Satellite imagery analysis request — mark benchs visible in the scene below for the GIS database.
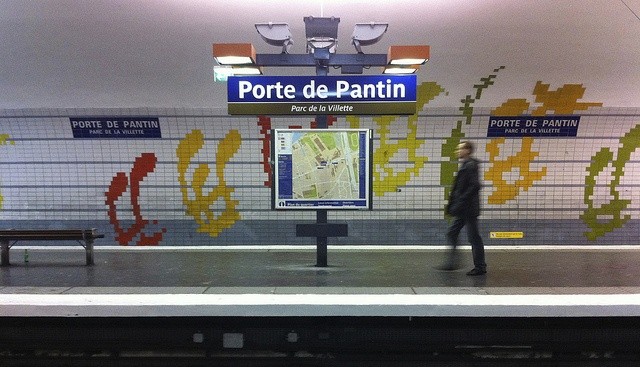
[0,228,105,265]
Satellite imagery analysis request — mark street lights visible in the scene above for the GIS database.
[213,42,428,268]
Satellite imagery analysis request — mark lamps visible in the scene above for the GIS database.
[351,21,389,53]
[387,45,431,65]
[213,64,263,81]
[254,23,293,52]
[213,43,256,65]
[382,64,419,74]
[303,16,341,54]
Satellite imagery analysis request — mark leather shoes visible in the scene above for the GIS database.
[467,269,487,276]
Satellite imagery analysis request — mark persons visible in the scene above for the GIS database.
[434,140,488,276]
